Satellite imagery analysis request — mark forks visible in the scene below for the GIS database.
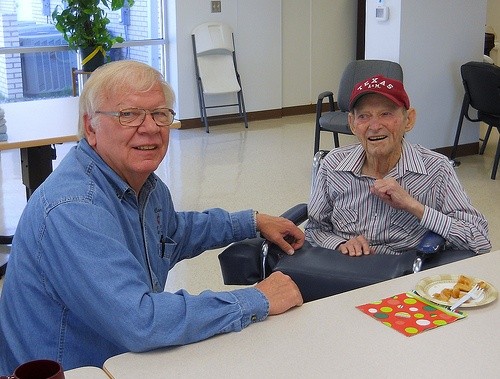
[450,284,484,311]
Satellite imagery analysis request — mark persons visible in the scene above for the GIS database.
[0,59,304,376]
[304,75,492,256]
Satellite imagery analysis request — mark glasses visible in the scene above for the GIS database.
[95,107,177,127]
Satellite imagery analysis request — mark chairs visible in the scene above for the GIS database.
[192,23,249,133]
[314,60,404,155]
[452,60,500,179]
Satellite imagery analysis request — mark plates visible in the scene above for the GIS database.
[415,274,499,308]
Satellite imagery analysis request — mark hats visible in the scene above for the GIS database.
[348,75,410,113]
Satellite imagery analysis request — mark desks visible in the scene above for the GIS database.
[0,96,181,281]
[102,248,500,379]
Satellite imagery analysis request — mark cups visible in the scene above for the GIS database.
[0,359,65,379]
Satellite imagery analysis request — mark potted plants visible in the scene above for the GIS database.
[52,0,136,71]
